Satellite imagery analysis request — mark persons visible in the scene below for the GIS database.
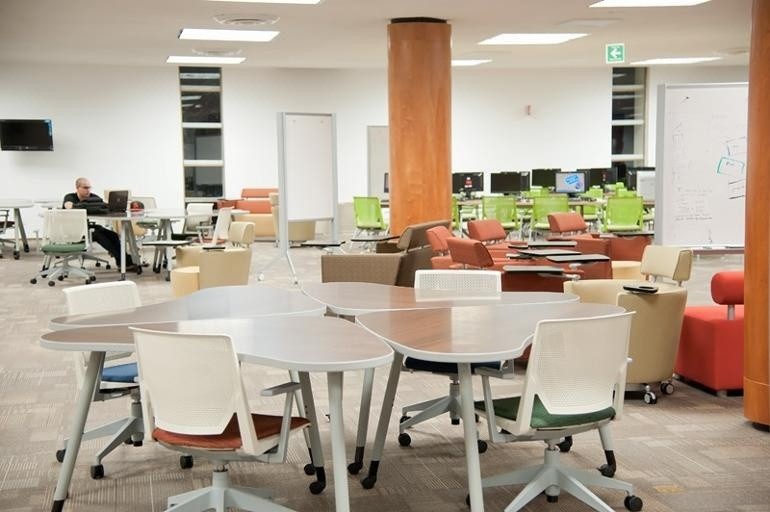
[61,178,132,271]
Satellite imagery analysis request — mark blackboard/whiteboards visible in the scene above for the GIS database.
[654,82,749,253]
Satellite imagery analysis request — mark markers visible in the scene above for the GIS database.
[702,246,713,249]
[725,246,745,248]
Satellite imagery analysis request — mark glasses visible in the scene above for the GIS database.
[77,184,93,190]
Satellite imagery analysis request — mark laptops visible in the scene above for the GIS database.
[98,190,129,214]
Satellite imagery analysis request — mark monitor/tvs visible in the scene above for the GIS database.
[577,169,617,193]
[384,173,389,193]
[501,171,530,191]
[453,172,484,200]
[0,119,55,152]
[555,171,586,194]
[490,172,521,195]
[637,171,656,199]
[626,167,655,189]
[532,168,561,188]
